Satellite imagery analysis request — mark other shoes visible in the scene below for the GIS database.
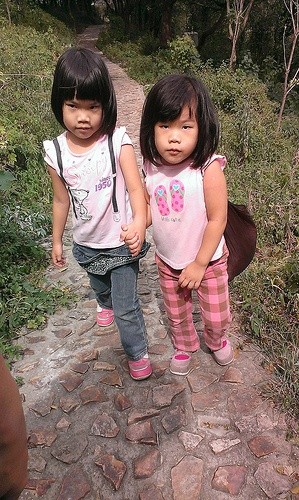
[211,339,234,366]
[170,349,192,375]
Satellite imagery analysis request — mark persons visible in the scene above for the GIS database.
[42,47,152,381]
[118,73,235,377]
[1,351,32,500]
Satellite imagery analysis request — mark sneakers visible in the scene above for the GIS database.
[127,351,152,380]
[96,303,114,326]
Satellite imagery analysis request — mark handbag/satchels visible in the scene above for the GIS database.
[224,200,258,282]
